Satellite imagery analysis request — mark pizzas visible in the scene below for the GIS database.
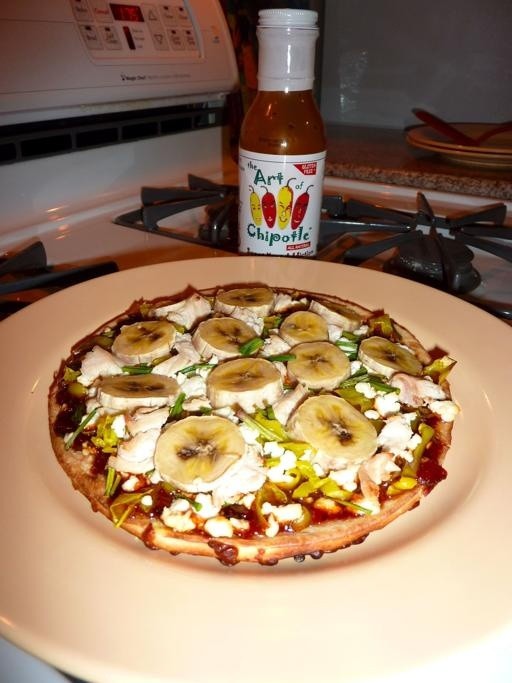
[47,281,461,569]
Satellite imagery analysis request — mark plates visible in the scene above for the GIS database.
[3,254,509,683]
[404,122,511,169]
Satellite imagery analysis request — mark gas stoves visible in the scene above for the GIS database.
[0,164,512,319]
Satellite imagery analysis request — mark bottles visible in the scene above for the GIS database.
[238,7,330,255]
